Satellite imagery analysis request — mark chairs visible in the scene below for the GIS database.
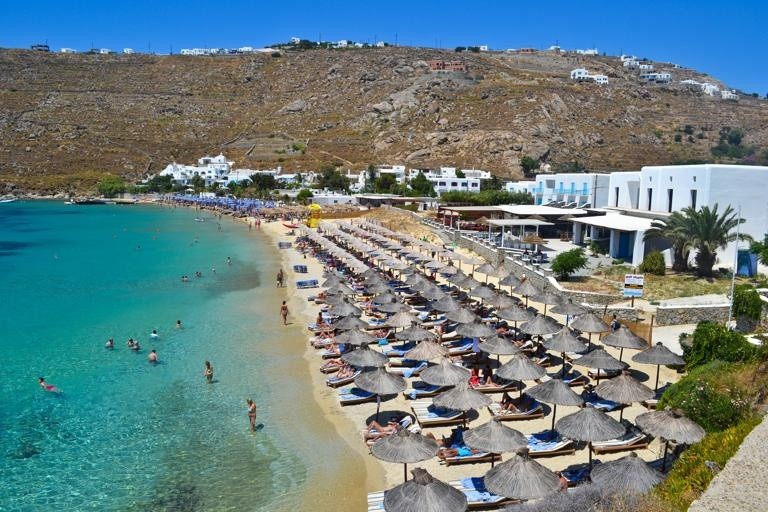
[296,272,680,511]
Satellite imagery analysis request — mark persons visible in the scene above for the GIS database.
[150,330,160,339]
[105,339,113,347]
[126,338,133,346]
[149,350,158,360]
[39,377,63,393]
[132,341,140,350]
[176,195,287,433]
